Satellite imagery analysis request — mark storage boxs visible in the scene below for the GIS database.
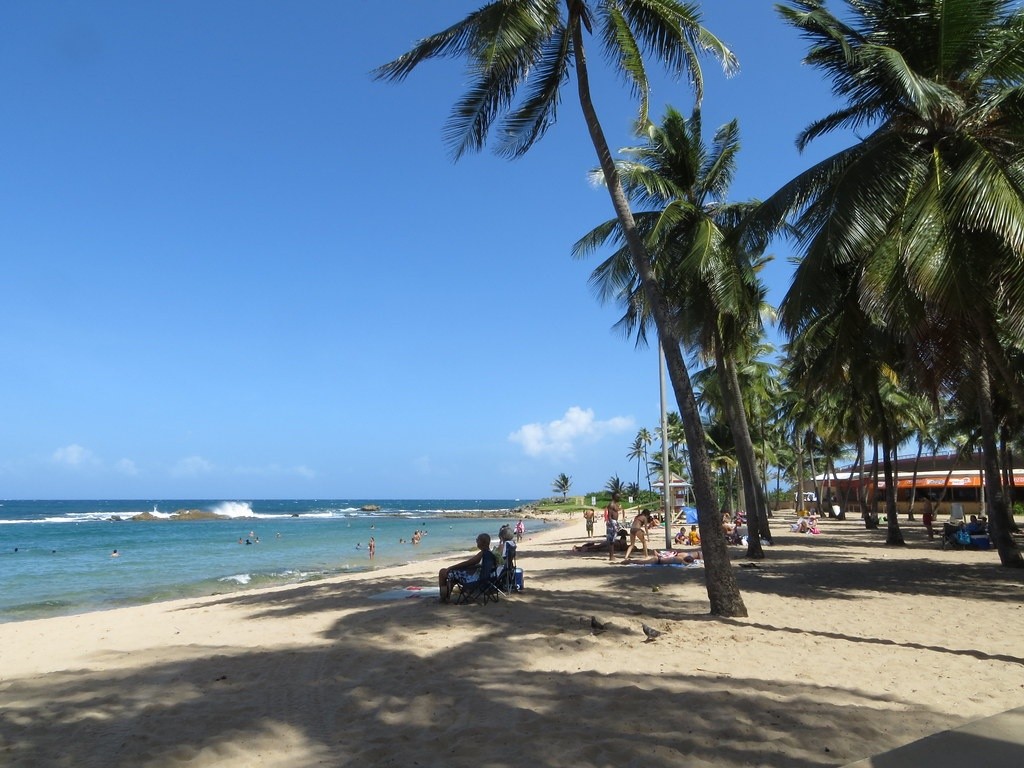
[970,535,989,549]
[513,568,524,590]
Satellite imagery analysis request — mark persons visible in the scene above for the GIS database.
[572,493,821,565]
[922,497,989,548]
[110,519,524,559]
[438,524,517,603]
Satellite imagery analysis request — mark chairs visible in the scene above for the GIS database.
[496,542,517,595]
[455,547,500,607]
[940,522,963,551]
[976,521,991,534]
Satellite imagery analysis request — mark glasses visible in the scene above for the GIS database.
[476,538,479,541]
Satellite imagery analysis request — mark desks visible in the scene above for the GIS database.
[848,504,872,513]
[880,504,900,515]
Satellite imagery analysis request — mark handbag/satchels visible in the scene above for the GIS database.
[514,568,524,590]
[956,525,970,545]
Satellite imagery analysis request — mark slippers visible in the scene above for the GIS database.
[405,594,420,598]
